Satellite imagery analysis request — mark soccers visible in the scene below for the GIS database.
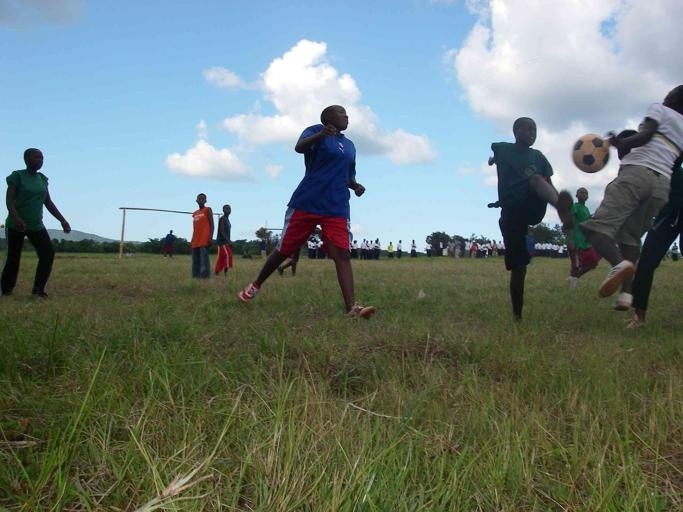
[573,134,609,173]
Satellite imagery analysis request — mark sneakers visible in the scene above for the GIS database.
[625,314,647,329]
[597,259,636,299]
[343,302,376,320]
[557,192,574,229]
[611,292,634,311]
[238,282,261,304]
[577,267,589,278]
[277,266,283,275]
[571,266,579,277]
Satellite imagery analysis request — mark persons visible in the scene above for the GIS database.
[1,148,71,297]
[672,243,679,261]
[487,117,574,323]
[238,105,377,319]
[566,188,602,280]
[387,242,393,258]
[437,238,505,258]
[410,240,417,257]
[308,232,381,261]
[214,205,233,276]
[579,84,683,311]
[191,193,214,278]
[264,232,282,255]
[525,230,535,263]
[279,207,301,275]
[164,230,174,258]
[397,240,402,258]
[618,131,683,329]
[533,241,570,258]
[426,241,433,257]
